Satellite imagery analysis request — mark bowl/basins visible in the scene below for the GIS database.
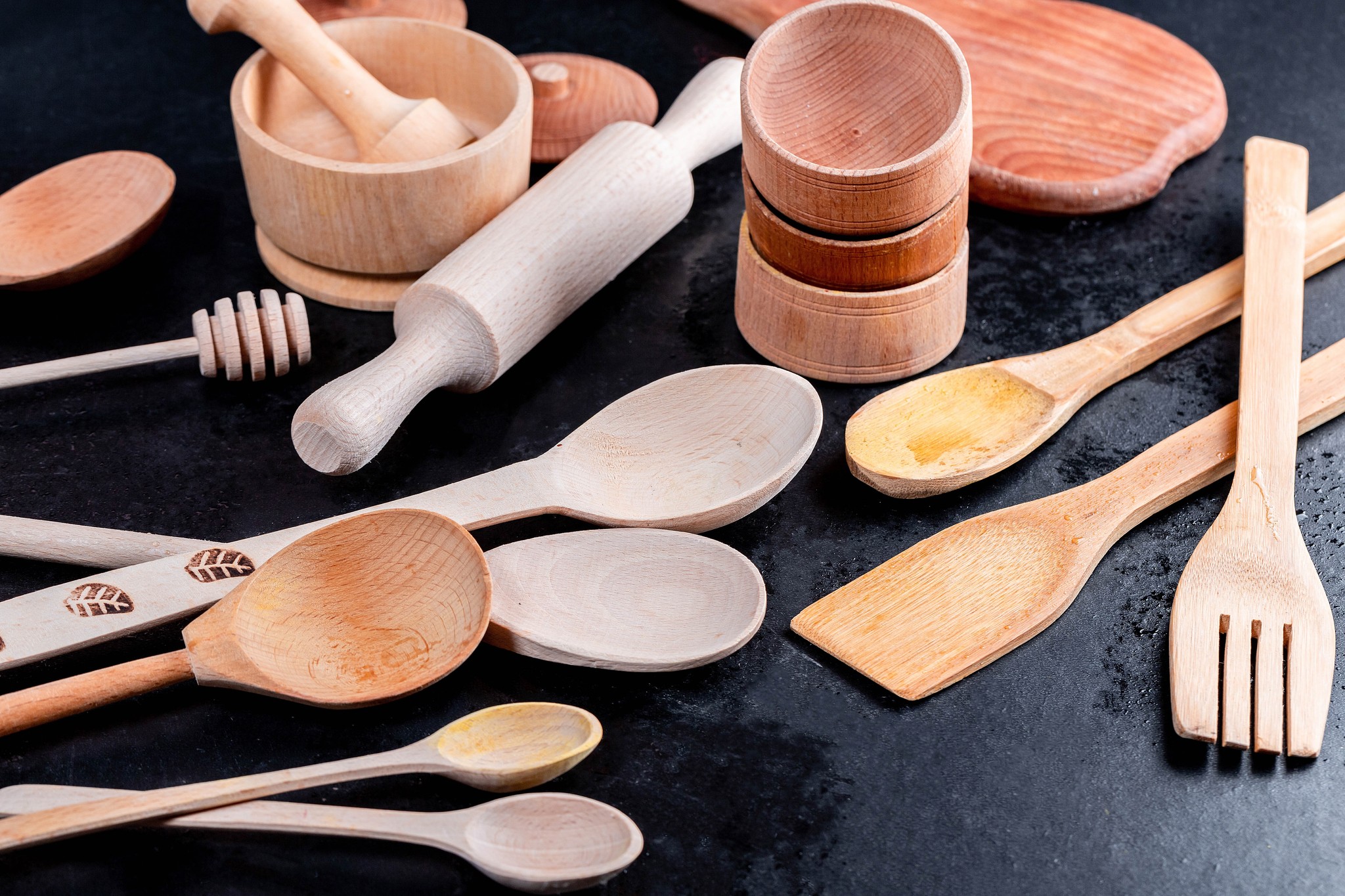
[228,16,534,275]
[735,1,972,384]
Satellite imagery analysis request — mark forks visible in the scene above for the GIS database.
[1170,133,1336,758]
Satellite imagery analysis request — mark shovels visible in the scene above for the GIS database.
[790,339,1345,703]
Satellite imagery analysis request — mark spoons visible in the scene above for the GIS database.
[843,187,1344,504]
[0,361,826,894]
